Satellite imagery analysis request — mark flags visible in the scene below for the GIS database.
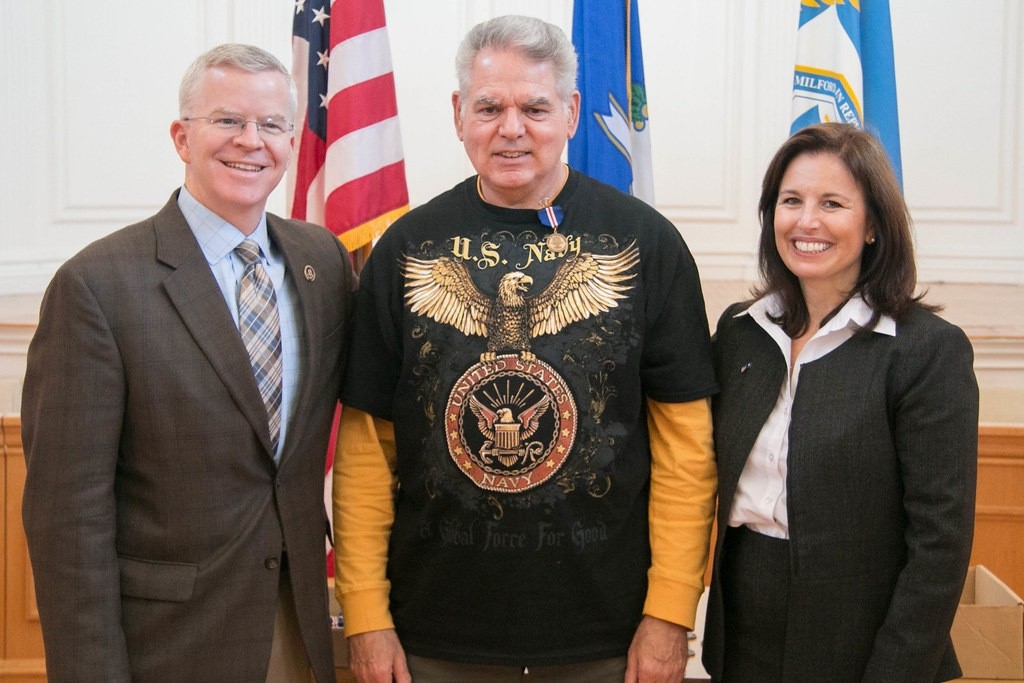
[790,0,905,198]
[568,0,655,208]
[284,0,410,272]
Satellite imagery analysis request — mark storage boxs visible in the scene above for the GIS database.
[949,565,1023,683]
[328,584,349,667]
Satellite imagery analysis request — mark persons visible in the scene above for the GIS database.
[701,123,980,683]
[20,42,355,683]
[331,16,729,683]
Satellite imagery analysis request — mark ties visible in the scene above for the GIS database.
[232,239,283,469]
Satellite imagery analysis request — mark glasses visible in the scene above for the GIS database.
[182,109,294,136]
